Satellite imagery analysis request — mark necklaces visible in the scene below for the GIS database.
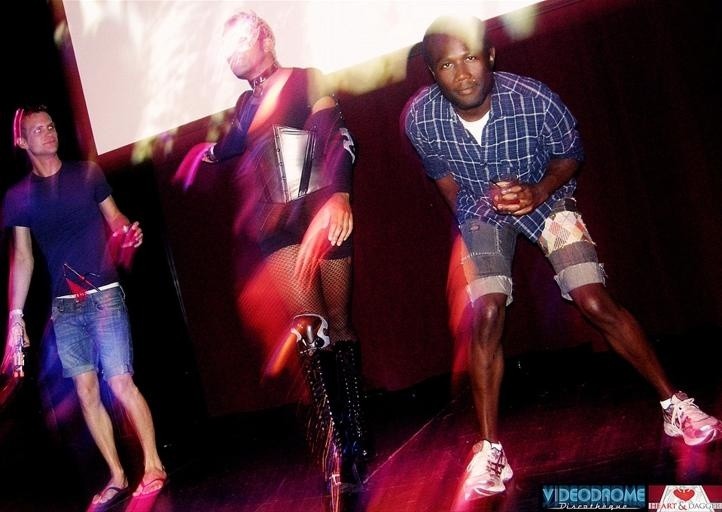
[250,60,281,98]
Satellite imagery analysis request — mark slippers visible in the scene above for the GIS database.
[93,485,129,506]
[135,471,166,499]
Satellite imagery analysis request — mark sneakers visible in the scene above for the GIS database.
[463,440,513,501]
[660,392,722,446]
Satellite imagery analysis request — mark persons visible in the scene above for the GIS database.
[183,8,369,512]
[397,4,721,505]
[2,101,167,509]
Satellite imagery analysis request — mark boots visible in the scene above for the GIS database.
[300,338,376,512]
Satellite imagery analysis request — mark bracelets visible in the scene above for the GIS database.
[203,145,217,162]
[8,308,24,319]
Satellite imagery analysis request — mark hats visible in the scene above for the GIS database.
[13,109,24,146]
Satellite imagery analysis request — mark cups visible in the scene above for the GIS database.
[488,173,519,215]
